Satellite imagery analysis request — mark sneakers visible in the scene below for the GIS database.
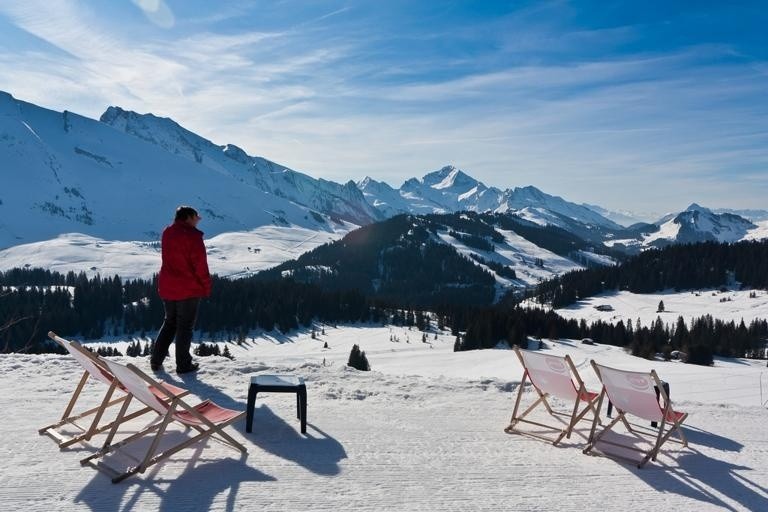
[151,362,162,371]
[175,362,200,375]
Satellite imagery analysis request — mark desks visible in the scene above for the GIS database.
[600,380,672,430]
[242,372,309,435]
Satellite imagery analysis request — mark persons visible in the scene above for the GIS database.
[150,204,214,375]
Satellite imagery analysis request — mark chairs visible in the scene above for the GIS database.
[502,341,602,447]
[80,350,247,482]
[579,356,691,469]
[32,328,207,454]
